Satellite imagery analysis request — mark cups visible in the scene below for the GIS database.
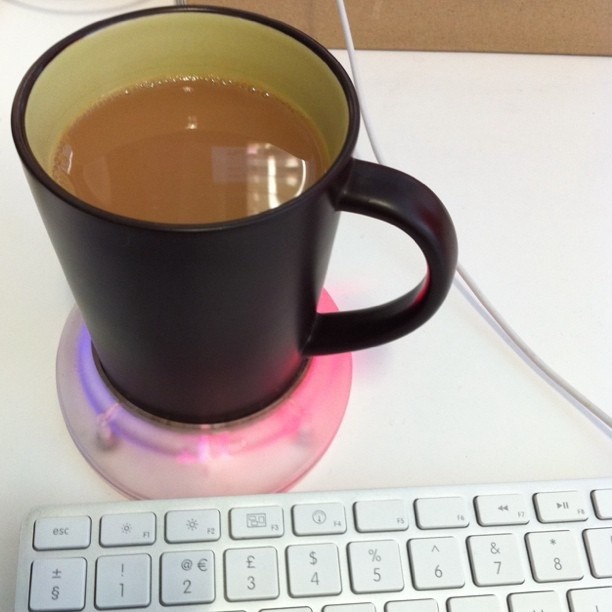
[12,5,456,422]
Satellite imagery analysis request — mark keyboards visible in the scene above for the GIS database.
[12,477,610,612]
